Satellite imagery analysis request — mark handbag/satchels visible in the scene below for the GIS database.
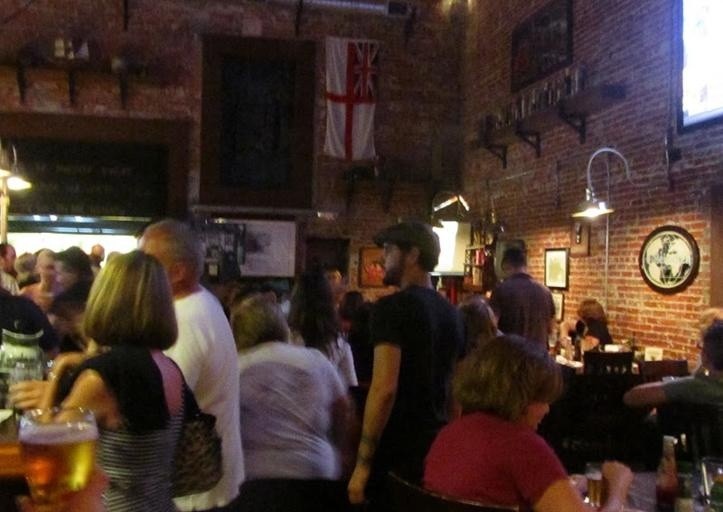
[168,414,222,498]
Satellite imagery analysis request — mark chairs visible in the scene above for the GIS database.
[366,468,525,511]
[541,342,722,475]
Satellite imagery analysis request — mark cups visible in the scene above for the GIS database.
[10,358,43,383]
[586,458,609,508]
[701,456,723,510]
[15,406,102,502]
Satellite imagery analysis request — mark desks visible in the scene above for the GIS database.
[583,467,721,512]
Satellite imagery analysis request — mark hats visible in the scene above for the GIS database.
[373,222,439,259]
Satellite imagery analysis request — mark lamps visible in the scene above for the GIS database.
[426,190,499,249]
[570,146,668,220]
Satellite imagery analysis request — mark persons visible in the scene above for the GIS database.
[621,308,723,473]
[203,220,635,510]
[2,219,247,510]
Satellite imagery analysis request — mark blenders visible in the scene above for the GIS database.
[201,217,247,274]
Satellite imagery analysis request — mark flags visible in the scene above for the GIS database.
[325,35,380,163]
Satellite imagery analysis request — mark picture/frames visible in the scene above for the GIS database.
[637,225,700,295]
[356,244,389,289]
[505,0,571,94]
[196,30,318,213]
[542,246,570,291]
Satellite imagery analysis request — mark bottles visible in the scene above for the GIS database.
[549,329,581,362]
[674,430,697,508]
[655,434,681,510]
[485,63,583,132]
